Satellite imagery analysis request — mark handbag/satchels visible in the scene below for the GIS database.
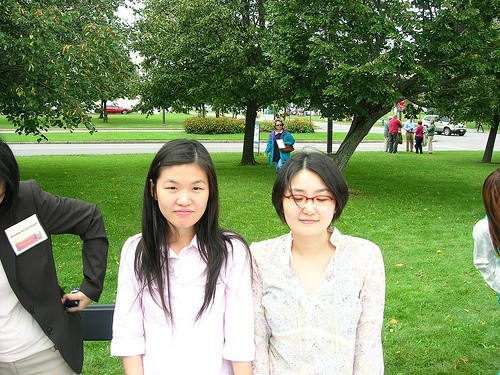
[281,145,294,152]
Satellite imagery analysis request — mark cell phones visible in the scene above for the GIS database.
[64,292,79,307]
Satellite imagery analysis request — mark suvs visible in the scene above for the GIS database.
[422,115,466,136]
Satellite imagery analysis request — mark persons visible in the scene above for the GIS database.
[249,151,386,375]
[0,139,109,375]
[411,120,424,154]
[473,168,500,294]
[388,114,402,153]
[264,120,295,175]
[404,116,414,153]
[110,139,255,375]
[476,122,482,131]
[426,118,436,154]
[384,117,393,152]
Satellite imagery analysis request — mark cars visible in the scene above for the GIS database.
[95,105,132,115]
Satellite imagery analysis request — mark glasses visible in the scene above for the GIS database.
[276,125,282,126]
[282,194,335,206]
